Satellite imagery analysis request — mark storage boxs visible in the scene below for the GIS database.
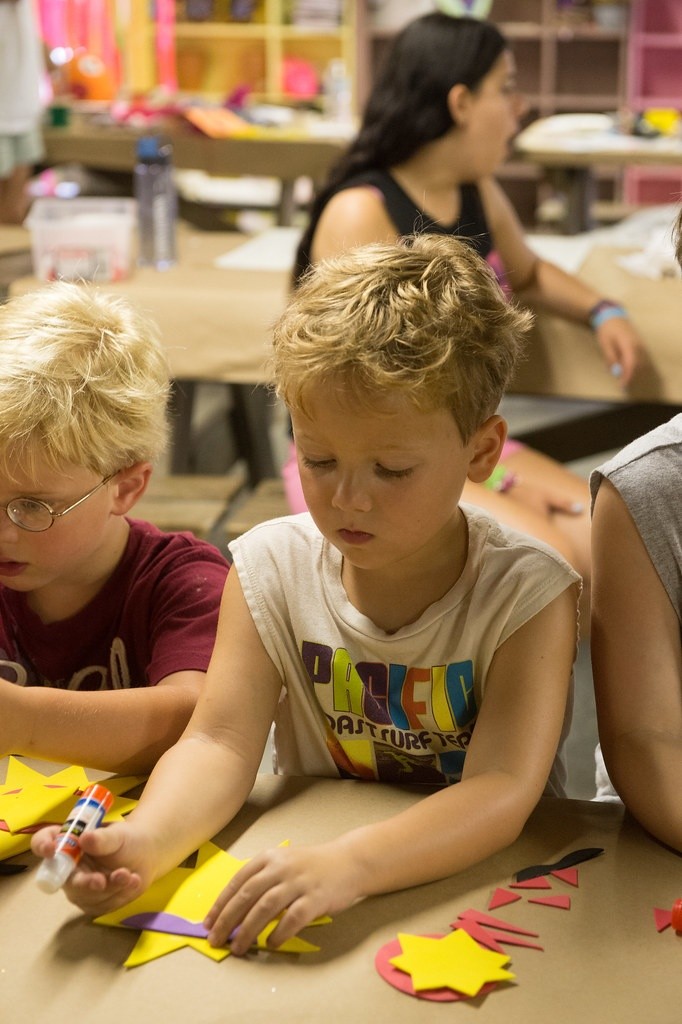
[23,197,141,283]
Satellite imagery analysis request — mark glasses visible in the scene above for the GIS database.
[0,470,122,532]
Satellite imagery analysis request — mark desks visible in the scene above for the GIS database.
[43,130,344,227]
[6,229,682,489]
[529,148,682,235]
[0,754,682,1024]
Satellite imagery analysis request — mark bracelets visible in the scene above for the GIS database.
[587,300,628,329]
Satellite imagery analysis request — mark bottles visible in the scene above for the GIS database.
[132,134,174,270]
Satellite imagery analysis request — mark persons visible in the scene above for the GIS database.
[0,282,230,776]
[588,412,682,856]
[29,234,584,956]
[264,13,637,626]
